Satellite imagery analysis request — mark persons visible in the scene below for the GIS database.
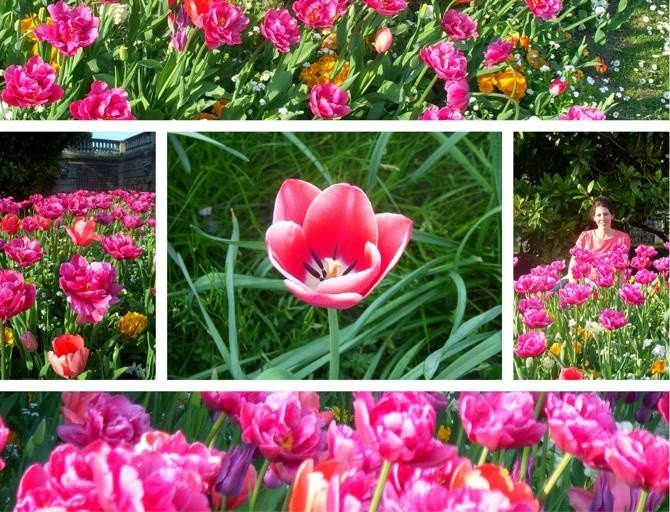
[537,198,631,299]
